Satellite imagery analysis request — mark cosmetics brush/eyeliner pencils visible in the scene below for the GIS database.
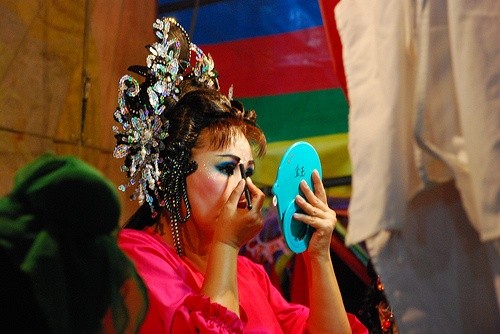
[238,164,253,210]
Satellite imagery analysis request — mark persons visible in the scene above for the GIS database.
[104,17,353,334]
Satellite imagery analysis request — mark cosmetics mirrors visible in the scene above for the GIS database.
[271,140,322,255]
